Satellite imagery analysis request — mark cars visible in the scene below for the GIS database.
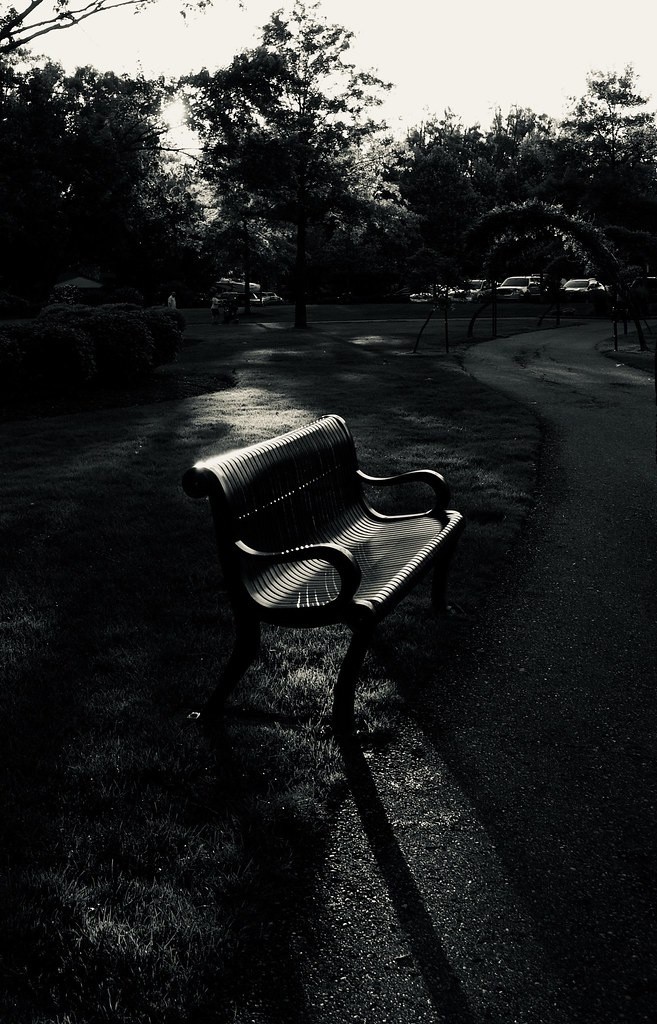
[629,276,657,303]
[219,290,283,308]
[379,285,411,303]
[409,283,452,304]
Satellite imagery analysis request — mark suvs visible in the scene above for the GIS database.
[496,276,547,300]
[561,277,607,291]
[448,279,496,304]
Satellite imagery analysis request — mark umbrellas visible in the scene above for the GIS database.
[54,277,103,304]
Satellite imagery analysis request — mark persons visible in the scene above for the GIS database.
[211,292,223,323]
[167,291,177,310]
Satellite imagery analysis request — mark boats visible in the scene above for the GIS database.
[214,276,260,292]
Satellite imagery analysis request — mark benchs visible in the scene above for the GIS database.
[180,414,465,741]
